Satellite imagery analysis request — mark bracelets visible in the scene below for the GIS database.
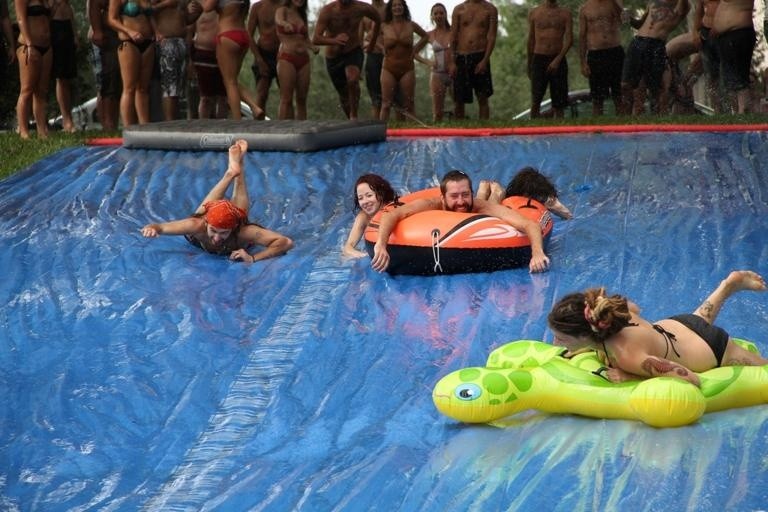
[251,255,255,263]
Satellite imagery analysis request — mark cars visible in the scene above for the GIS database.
[502,84,719,124]
[52,92,271,133]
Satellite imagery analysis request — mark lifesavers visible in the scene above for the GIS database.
[432,337,764,424]
[366,187,554,274]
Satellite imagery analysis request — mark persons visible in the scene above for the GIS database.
[343,174,573,258]
[547,270,767,387]
[247,0,498,119]
[1,0,250,139]
[139,138,294,262]
[506,166,559,210]
[528,0,766,118]
[370,170,549,275]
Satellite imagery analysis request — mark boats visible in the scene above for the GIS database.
[360,173,556,269]
